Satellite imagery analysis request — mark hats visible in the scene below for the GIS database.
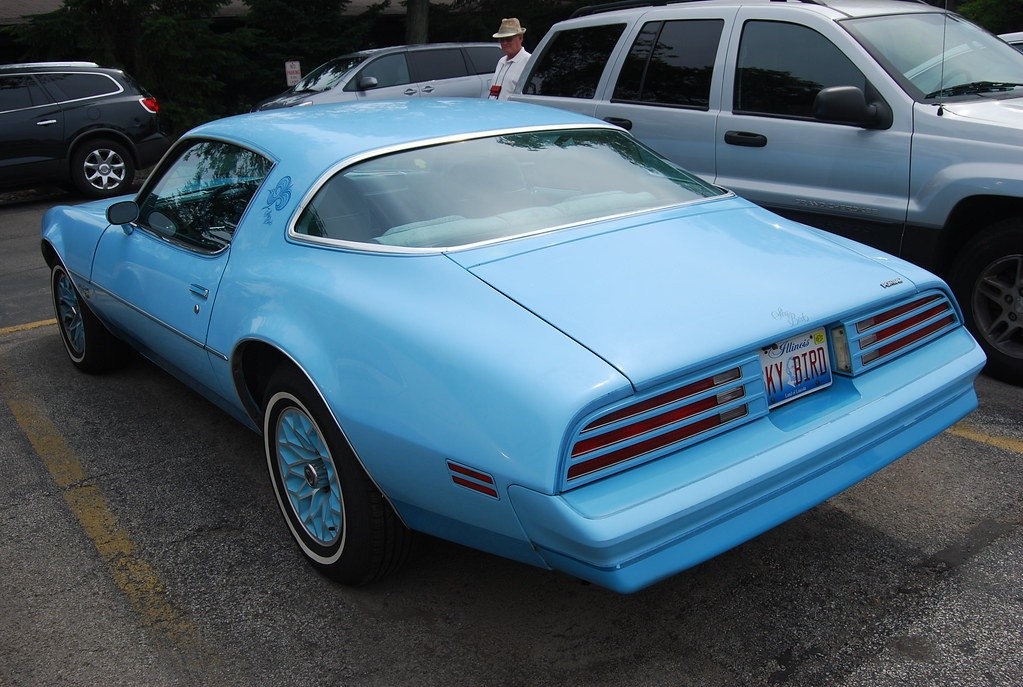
[492,18,526,38]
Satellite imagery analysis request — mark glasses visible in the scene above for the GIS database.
[498,35,521,43]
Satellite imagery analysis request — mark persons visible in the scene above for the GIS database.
[488,18,531,101]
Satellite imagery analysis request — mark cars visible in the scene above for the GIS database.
[38,91,989,591]
[902,31,1023,99]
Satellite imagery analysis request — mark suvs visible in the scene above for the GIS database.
[249,38,507,113]
[0,60,172,200]
[507,0,1023,387]
